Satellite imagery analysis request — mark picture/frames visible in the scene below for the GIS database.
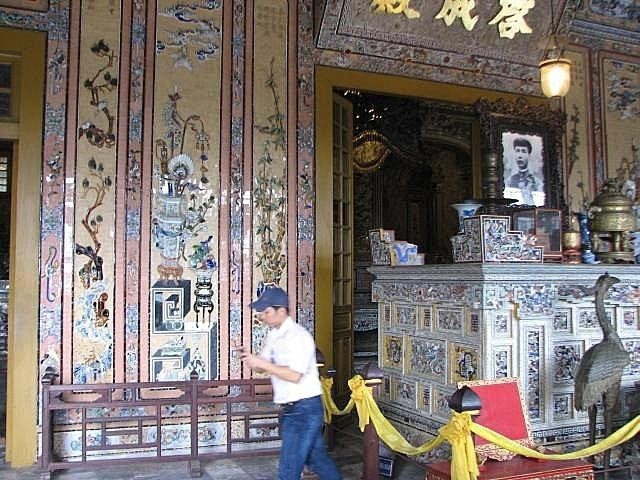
[513,208,563,255]
[477,94,570,234]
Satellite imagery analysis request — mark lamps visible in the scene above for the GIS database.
[539,0,572,114]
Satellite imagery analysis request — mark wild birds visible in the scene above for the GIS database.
[573,274,631,480]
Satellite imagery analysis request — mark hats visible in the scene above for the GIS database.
[247,288,289,313]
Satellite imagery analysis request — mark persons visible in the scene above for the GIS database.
[507,135,542,190]
[230,287,346,479]
